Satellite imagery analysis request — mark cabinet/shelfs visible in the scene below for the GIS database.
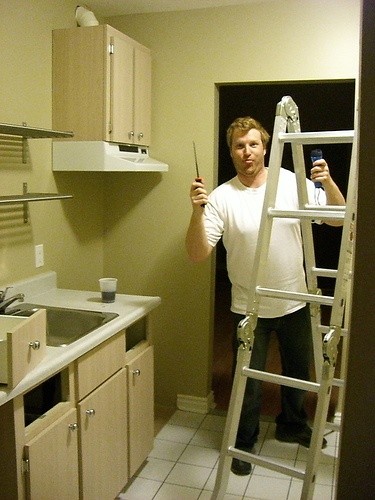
[0,313,155,500]
[50,23,156,146]
[0,122,74,226]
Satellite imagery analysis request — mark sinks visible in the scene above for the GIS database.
[11,303,120,347]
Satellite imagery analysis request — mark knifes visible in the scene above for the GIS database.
[193,140,206,206]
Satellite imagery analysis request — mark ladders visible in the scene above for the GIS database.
[210,93,362,498]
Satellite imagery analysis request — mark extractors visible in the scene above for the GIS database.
[52,141,168,173]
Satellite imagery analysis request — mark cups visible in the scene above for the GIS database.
[99,277,118,302]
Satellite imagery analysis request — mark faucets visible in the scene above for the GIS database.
[0,287,24,315]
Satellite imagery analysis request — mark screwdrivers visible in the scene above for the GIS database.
[192,140,206,208]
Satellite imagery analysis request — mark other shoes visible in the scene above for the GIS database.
[231,443,252,474]
[274,422,328,449]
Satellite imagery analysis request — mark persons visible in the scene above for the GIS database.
[185,117,346,474]
